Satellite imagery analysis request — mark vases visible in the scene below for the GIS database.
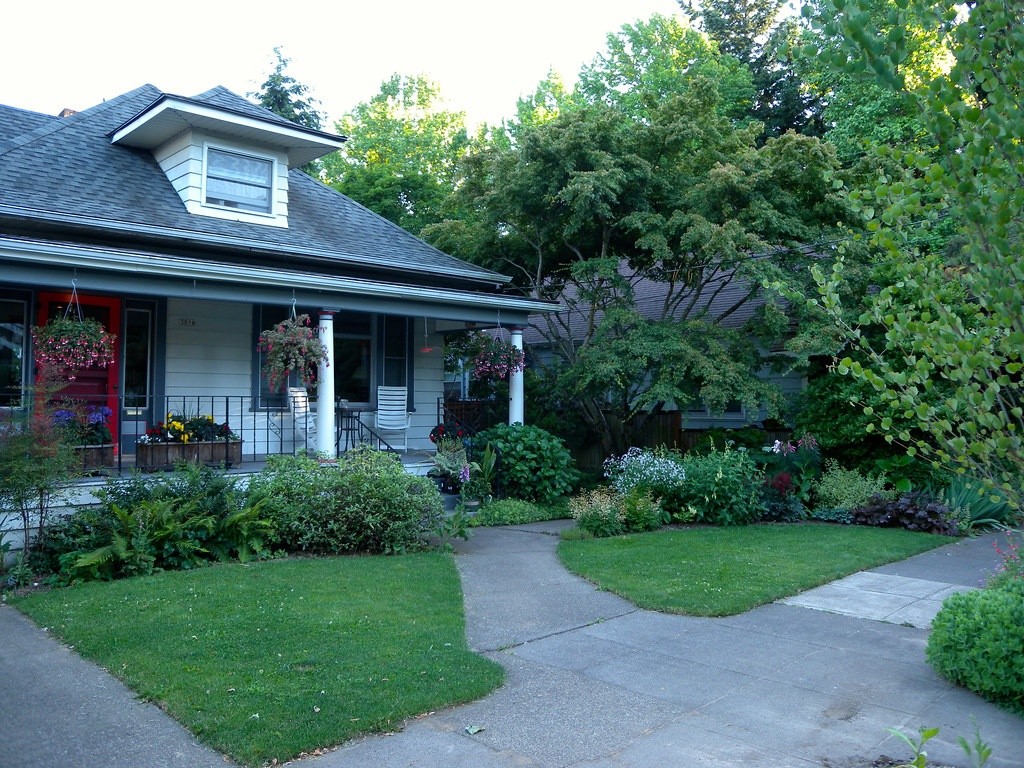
[134,439,244,473]
[58,447,114,477]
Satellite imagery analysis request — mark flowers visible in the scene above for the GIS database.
[51,404,112,446]
[474,337,526,380]
[134,400,239,447]
[256,314,330,395]
[30,312,116,381]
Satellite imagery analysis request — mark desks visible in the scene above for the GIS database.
[310,407,378,456]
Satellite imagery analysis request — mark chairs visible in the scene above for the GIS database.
[288,387,341,456]
[374,386,412,454]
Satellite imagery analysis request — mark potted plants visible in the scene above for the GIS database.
[421,438,467,495]
[459,442,497,512]
